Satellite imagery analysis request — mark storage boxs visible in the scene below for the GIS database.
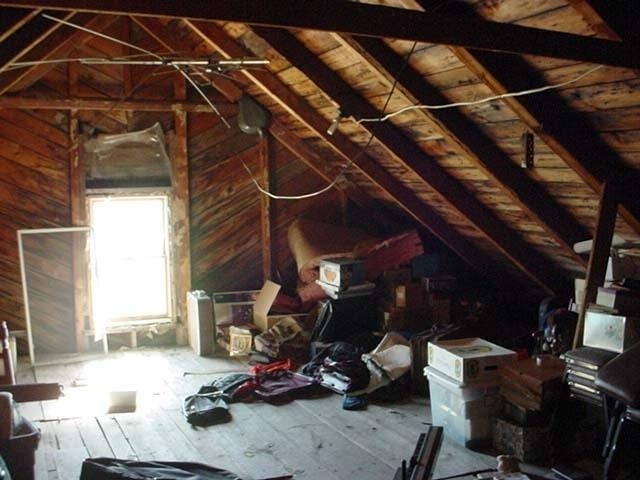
[318,256,366,288]
[427,337,518,383]
[424,365,500,447]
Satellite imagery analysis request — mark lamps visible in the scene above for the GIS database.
[326,109,349,135]
